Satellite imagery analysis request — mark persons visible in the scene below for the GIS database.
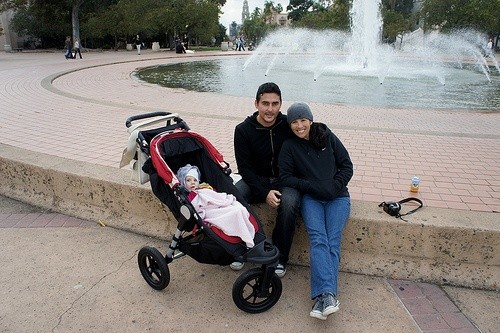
[135,35,142,55]
[483,39,496,58]
[65,36,82,59]
[249,41,255,50]
[229,83,300,277]
[278,102,354,320]
[175,34,189,54]
[178,164,248,237]
[235,33,246,51]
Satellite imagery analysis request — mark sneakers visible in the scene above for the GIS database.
[229,260,245,271]
[274,264,287,278]
[310,292,341,321]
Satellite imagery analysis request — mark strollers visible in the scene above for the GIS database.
[125,111,283,314]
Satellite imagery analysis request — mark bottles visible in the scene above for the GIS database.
[410,177,420,192]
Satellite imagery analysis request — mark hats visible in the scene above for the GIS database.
[287,103,313,124]
[186,168,199,182]
